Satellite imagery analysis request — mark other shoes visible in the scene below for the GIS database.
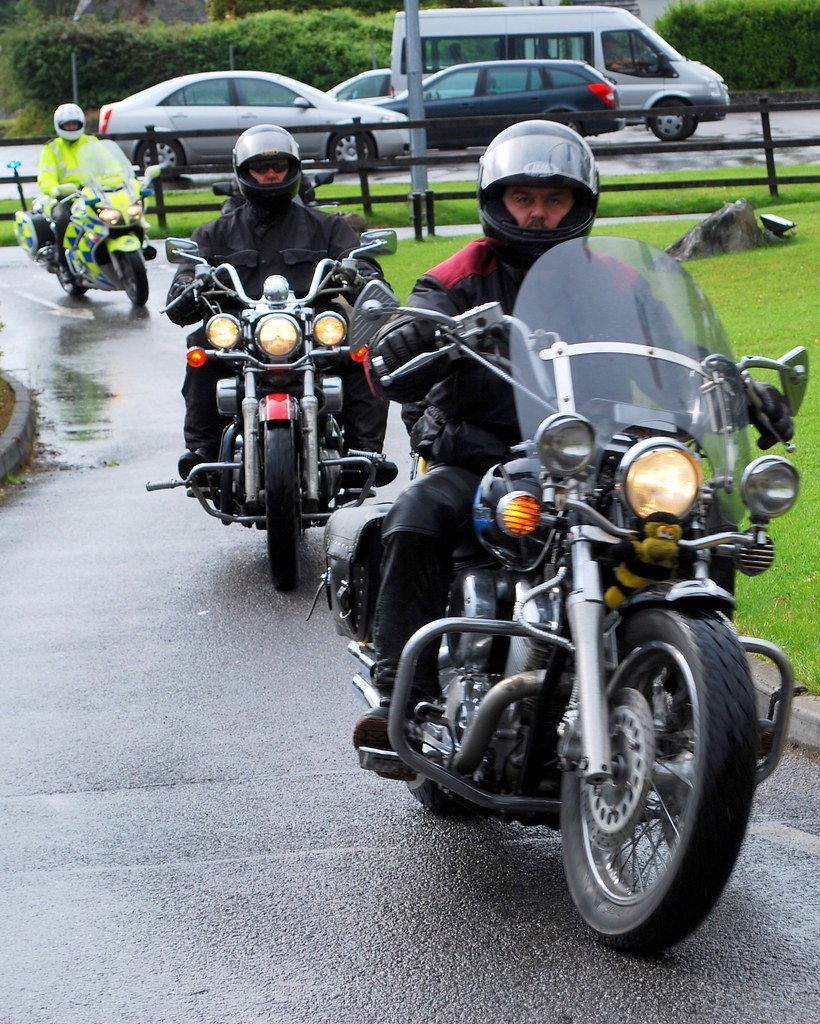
[60,264,73,284]
[179,448,218,487]
[353,706,417,782]
[343,454,398,488]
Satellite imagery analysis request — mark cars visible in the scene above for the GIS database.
[98,70,412,176]
[322,68,392,106]
[368,58,625,154]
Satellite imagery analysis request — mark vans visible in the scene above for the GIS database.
[390,6,730,142]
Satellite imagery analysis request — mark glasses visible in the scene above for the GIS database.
[247,161,289,175]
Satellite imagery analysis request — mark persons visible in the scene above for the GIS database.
[37,103,126,285]
[165,123,397,500]
[351,118,796,780]
[219,171,317,215]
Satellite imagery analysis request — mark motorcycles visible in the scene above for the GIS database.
[13,138,163,307]
[341,301,811,958]
[160,228,403,593]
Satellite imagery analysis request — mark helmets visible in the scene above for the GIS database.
[233,124,302,210]
[53,103,86,141]
[476,120,601,270]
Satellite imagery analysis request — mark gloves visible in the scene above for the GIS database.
[368,313,447,385]
[746,383,794,451]
[165,283,207,328]
[345,261,394,309]
